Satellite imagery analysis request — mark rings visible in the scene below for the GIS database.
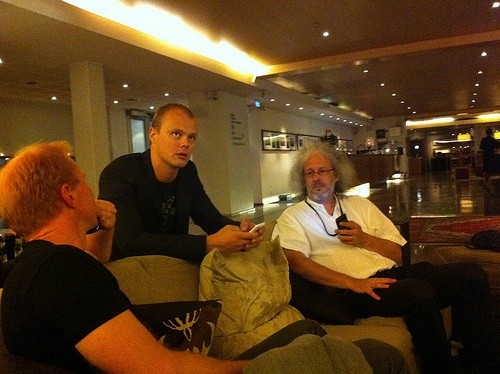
[349,236,352,241]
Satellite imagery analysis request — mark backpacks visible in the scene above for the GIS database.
[464,229,500,253]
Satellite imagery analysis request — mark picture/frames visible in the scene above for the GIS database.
[261,129,353,153]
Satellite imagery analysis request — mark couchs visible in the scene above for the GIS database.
[102,255,450,374]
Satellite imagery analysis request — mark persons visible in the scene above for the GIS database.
[480,129,499,176]
[270,140,500,374]
[97,103,268,265]
[0,140,251,374]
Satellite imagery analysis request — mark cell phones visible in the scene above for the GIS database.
[248,221,266,233]
[336,214,349,236]
[86,225,101,235]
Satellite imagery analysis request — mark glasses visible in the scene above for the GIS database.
[303,168,335,177]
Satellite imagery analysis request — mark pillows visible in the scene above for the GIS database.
[199,235,304,358]
[131,299,222,356]
